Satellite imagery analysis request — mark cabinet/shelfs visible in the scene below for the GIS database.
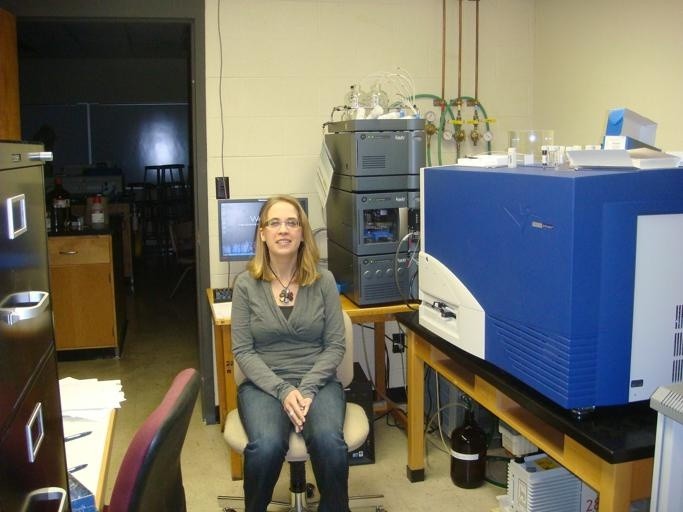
[0,139,72,512]
[46,199,134,357]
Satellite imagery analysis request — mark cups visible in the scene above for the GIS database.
[63,212,84,235]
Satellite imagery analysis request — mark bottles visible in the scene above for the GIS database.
[91,191,105,233]
[339,81,422,122]
[48,173,71,234]
[450,408,488,489]
[498,132,558,170]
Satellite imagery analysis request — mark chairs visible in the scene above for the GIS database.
[167,221,195,299]
[217,310,386,512]
[101,369,204,512]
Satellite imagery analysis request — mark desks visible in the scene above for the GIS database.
[206,287,426,480]
[59,378,118,512]
[394,312,659,512]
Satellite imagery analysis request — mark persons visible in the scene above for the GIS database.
[230,196,349,511]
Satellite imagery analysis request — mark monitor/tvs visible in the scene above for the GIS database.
[218,198,308,262]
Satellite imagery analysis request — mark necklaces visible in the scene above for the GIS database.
[266,263,298,303]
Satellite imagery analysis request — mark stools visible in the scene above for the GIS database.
[127,164,191,248]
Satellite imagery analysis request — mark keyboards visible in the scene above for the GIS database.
[214,287,232,302]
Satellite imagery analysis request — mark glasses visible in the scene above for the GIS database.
[263,218,301,228]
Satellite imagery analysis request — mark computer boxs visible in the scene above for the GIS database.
[344,362,375,465]
